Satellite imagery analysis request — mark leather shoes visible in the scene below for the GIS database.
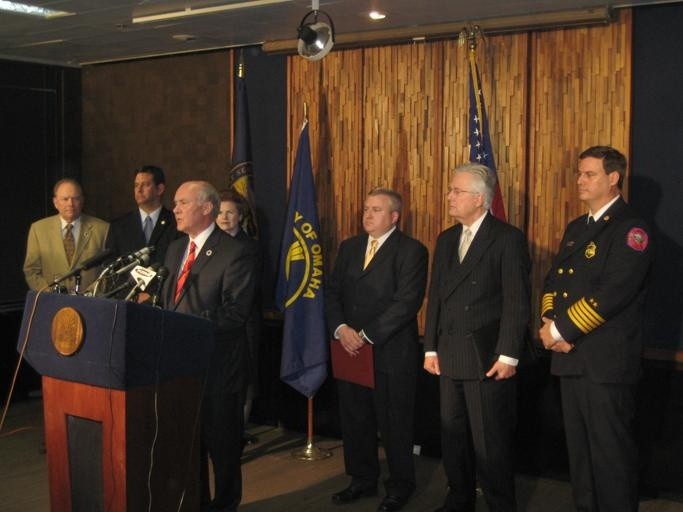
[378,495,410,512]
[331,480,378,505]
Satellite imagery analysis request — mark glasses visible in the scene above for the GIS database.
[448,187,474,196]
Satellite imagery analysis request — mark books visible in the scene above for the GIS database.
[330,338,376,389]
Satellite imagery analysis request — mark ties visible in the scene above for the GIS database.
[143,215,154,244]
[64,224,76,267]
[363,239,379,269]
[587,216,595,227]
[174,241,196,305]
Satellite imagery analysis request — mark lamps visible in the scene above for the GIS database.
[297,1,336,61]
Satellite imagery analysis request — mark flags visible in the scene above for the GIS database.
[227,79,258,240]
[277,122,328,397]
[467,57,508,223]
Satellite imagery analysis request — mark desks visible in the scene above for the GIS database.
[17,289,217,511]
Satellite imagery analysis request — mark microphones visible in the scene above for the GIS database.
[126,260,162,300]
[114,252,151,277]
[153,265,170,304]
[126,242,158,262]
[103,275,136,298]
[53,247,116,285]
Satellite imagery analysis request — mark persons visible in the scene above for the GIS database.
[155,179,259,512]
[23,178,112,455]
[216,189,277,423]
[327,188,430,512]
[109,164,188,266]
[537,144,654,512]
[421,162,533,512]
